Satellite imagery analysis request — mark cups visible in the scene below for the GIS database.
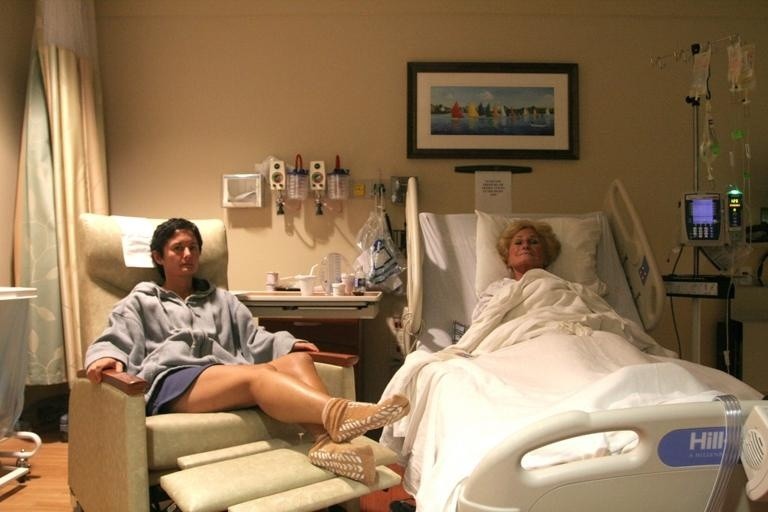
[341,273,355,297]
[298,276,313,298]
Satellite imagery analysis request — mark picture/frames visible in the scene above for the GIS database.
[406,60,580,160]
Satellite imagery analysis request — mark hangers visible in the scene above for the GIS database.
[647,32,738,366]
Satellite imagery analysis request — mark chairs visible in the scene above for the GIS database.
[67,214,403,512]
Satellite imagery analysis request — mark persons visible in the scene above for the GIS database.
[473,218,561,324]
[84,218,411,487]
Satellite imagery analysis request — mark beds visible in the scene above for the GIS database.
[404,176,768,511]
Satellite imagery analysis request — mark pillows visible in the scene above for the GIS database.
[473,208,611,300]
